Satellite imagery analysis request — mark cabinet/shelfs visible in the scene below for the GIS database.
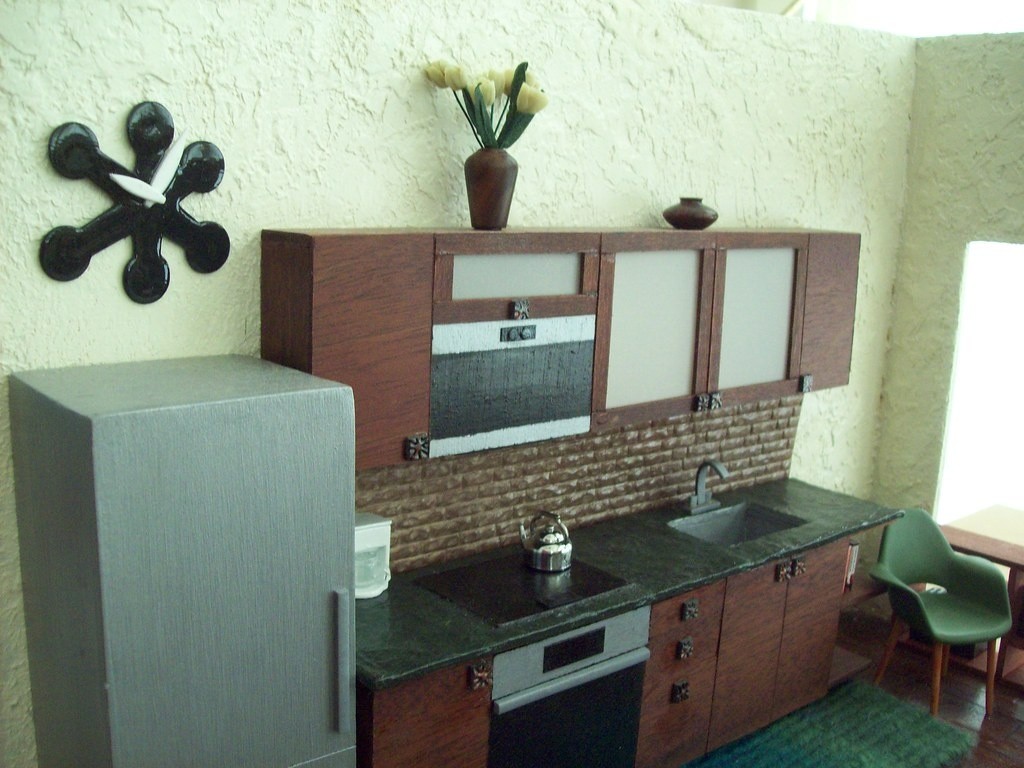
[706,535,851,755]
[356,650,494,768]
[262,223,863,474]
[631,577,727,768]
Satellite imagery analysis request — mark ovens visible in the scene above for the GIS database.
[485,605,651,768]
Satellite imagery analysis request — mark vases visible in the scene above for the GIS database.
[663,197,719,230]
[463,148,518,231]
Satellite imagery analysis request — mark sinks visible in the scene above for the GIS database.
[665,501,813,548]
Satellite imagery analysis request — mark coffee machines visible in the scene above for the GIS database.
[354,513,391,599]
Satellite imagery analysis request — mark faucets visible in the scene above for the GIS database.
[682,456,730,515]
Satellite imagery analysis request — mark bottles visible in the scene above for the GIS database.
[663,197,719,230]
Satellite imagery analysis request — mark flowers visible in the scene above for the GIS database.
[426,58,550,148]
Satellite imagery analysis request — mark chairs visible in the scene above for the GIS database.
[868,508,1011,716]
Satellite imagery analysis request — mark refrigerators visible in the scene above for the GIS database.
[6,350,358,768]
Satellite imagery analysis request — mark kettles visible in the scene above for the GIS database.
[518,509,573,572]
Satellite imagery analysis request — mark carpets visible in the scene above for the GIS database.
[676,677,978,768]
